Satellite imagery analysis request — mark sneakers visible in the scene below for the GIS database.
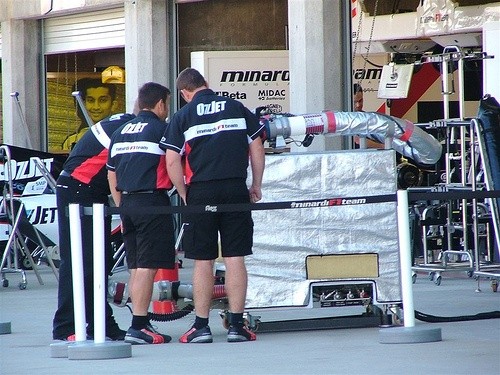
[124,323,171,345]
[179,324,212,343]
[227,325,256,342]
[86,315,127,340]
[54,329,75,342]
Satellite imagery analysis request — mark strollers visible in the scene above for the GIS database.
[4,199,57,270]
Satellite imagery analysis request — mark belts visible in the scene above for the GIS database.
[60,170,71,177]
[121,190,154,194]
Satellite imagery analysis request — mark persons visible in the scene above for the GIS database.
[51,94,140,340]
[353,83,386,150]
[159,68,268,344]
[62,80,118,151]
[106,82,181,344]
